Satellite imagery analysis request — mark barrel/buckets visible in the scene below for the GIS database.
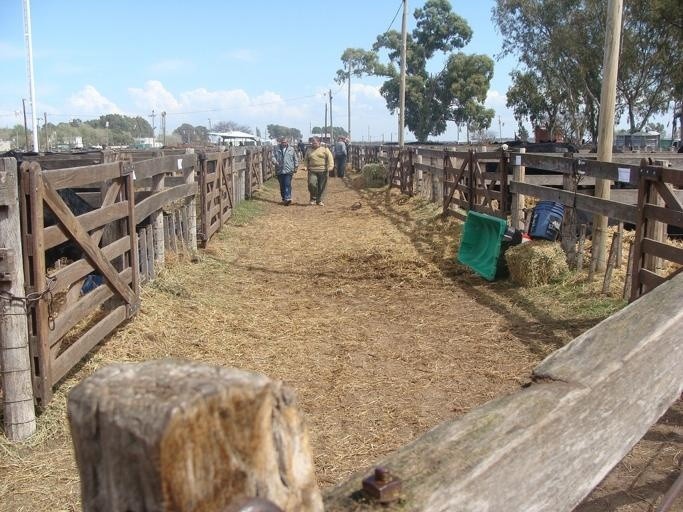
[527,200,564,242]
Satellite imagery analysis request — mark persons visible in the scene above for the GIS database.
[297,136,352,177]
[271,138,299,206]
[300,136,334,205]
